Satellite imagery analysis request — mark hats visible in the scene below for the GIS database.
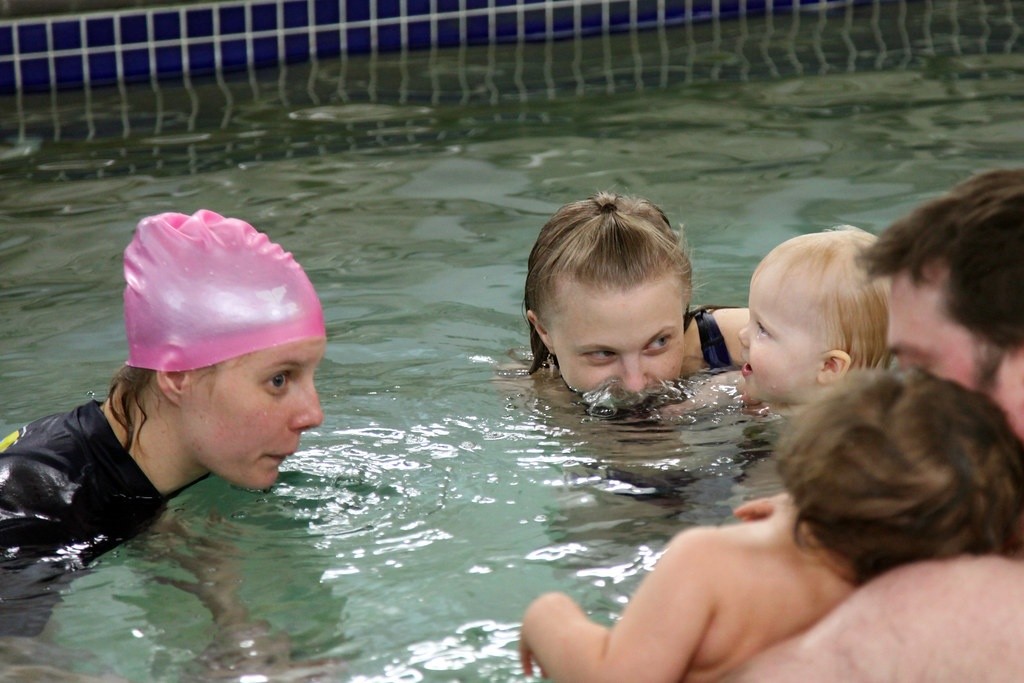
[123,208,326,372]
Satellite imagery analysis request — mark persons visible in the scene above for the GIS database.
[519,366,1024,683]
[737,219,893,423]
[716,166,1024,683]
[515,190,751,398]
[1,207,327,571]
